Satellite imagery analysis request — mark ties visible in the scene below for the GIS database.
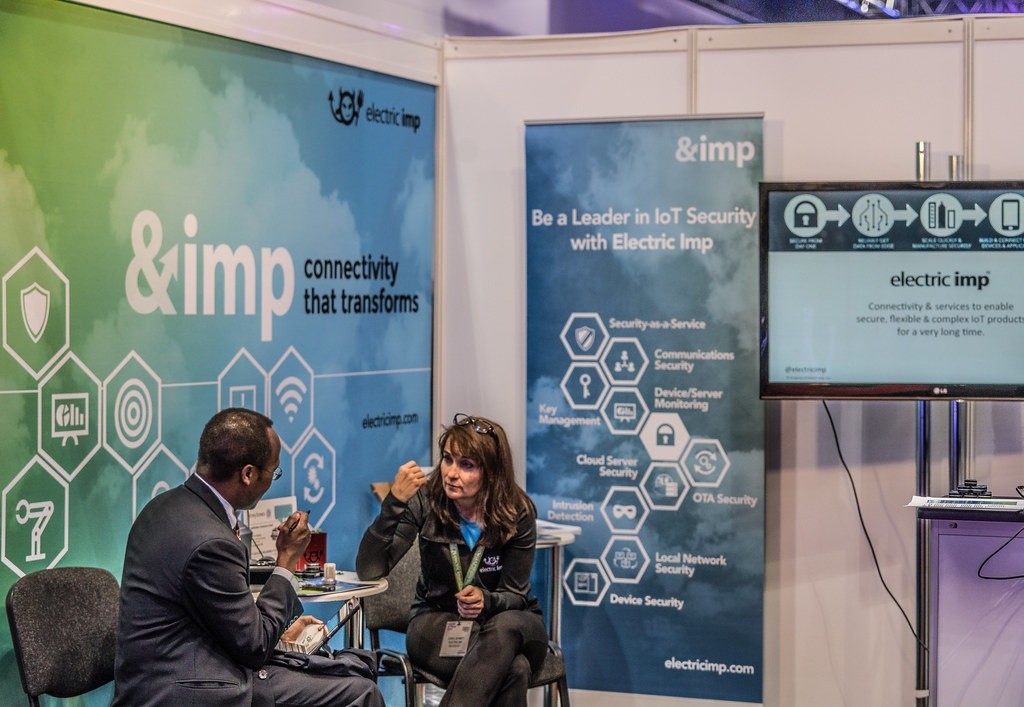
[233,522,241,540]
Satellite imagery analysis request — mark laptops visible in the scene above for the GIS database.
[272,596,361,656]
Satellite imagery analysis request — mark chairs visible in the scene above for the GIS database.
[5,567,121,707]
[358,532,571,707]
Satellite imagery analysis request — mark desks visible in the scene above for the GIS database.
[246,569,389,654]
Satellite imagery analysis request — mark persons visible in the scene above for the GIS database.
[356,413,549,707]
[112,407,388,707]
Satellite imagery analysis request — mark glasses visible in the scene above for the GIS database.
[261,467,283,480]
[453,413,500,447]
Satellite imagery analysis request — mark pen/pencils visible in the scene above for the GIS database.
[288,509,312,533]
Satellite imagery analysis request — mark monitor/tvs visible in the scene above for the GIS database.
[758,180,1024,401]
